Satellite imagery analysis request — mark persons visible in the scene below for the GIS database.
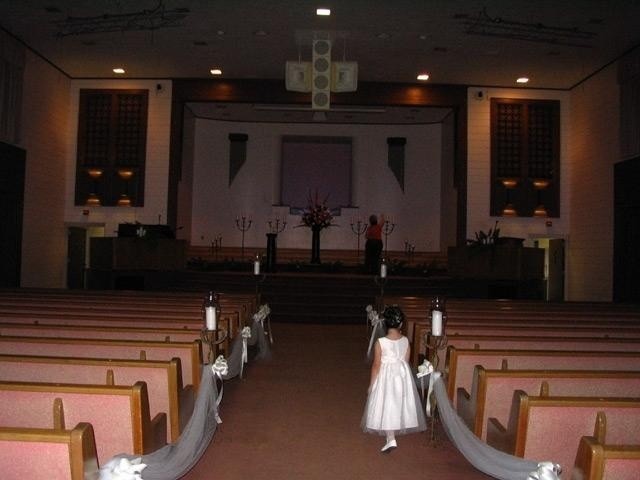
[363,214,385,275]
[360,306,428,454]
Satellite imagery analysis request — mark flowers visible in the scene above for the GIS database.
[292,188,344,231]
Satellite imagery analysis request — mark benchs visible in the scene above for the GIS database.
[1,280,262,480]
[380,297,640,480]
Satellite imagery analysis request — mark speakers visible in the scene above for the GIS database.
[155,83,162,91]
[477,90,483,99]
[312,38,330,110]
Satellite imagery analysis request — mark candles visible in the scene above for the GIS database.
[210,233,222,243]
[233,206,287,222]
[381,258,387,278]
[404,239,415,248]
[350,213,395,226]
[254,255,261,275]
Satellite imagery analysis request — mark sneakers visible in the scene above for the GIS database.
[380,439,398,454]
